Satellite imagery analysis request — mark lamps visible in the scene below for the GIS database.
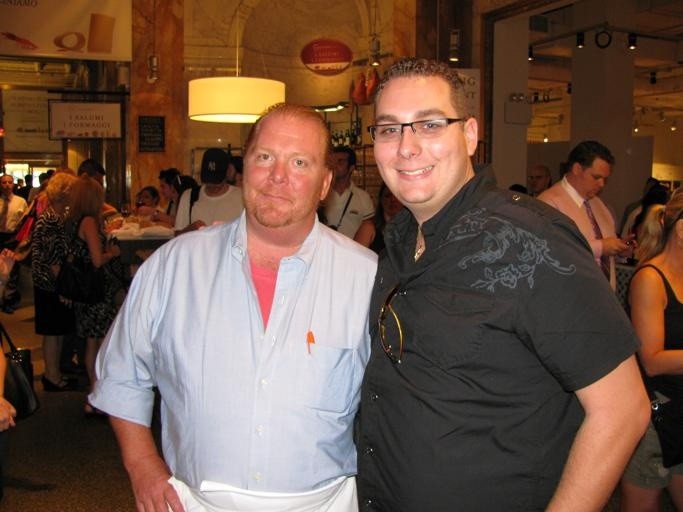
[186,0,286,124]
[526,24,639,61]
[444,1,463,63]
[368,0,383,67]
[312,103,346,113]
[146,1,160,81]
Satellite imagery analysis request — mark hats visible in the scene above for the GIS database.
[200,149,228,184]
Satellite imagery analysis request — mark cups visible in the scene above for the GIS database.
[120,203,130,219]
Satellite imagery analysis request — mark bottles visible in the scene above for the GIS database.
[330,129,365,148]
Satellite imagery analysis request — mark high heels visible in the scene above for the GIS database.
[41,376,73,392]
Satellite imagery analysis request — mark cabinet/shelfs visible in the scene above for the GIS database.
[352,141,382,200]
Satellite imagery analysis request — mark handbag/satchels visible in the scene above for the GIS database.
[55,265,106,304]
[5,349,41,420]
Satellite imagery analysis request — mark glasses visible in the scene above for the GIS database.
[377,283,407,363]
[367,119,466,143]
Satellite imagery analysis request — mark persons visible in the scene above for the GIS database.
[1,342,18,430]
[354,58,651,511]
[628,185,683,512]
[2,149,244,415]
[508,161,551,197]
[537,142,628,293]
[614,177,671,308]
[87,102,378,511]
[316,145,405,255]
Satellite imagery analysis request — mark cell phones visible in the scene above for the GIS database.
[625,239,632,246]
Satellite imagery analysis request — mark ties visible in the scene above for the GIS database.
[583,201,610,281]
